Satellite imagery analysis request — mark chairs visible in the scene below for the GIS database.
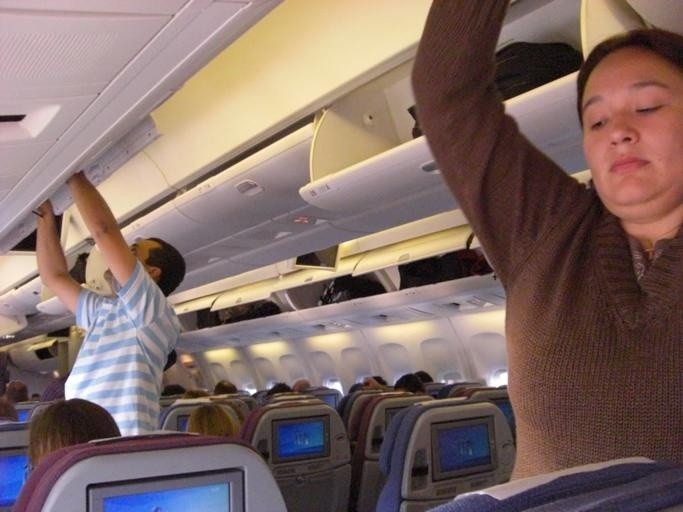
[1,381,682,512]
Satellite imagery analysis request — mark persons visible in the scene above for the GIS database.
[409,0,681,480]
[26,396,121,472]
[4,380,29,404]
[162,369,434,436]
[36,170,185,437]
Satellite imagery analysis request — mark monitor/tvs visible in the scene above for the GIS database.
[0,386,514,512]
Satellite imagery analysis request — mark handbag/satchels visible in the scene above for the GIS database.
[408,42,585,138]
[318,275,386,308]
[399,249,493,290]
[197,298,280,327]
